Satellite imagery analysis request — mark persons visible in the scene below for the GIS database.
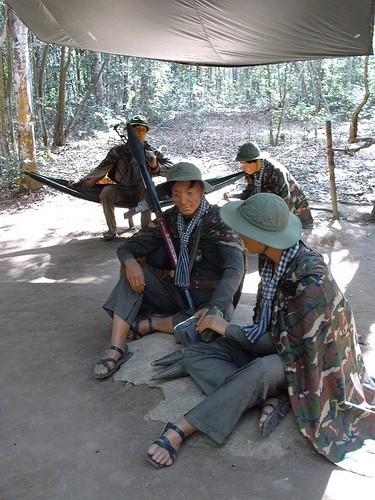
[93,162,248,379]
[146,193,375,479]
[223,141,314,225]
[69,115,174,239]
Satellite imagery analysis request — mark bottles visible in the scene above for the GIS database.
[201,305,223,342]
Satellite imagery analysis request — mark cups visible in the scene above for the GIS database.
[173,317,203,346]
